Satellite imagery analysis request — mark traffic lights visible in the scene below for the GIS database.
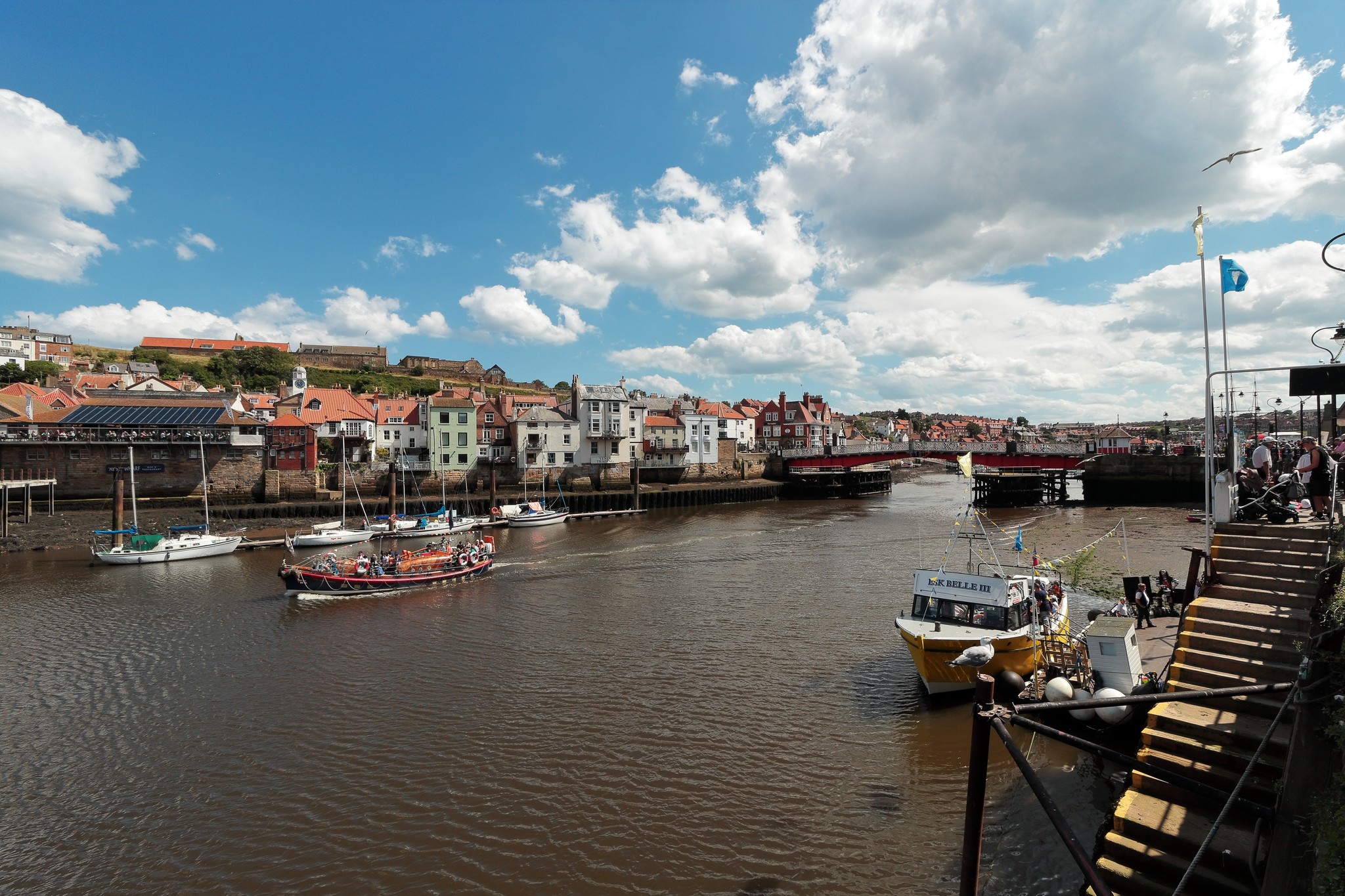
[1166,426,1170,435]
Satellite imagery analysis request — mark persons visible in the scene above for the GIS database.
[1187,441,1191,445]
[1215,442,1226,456]
[994,574,1001,577]
[440,539,487,565]
[1134,583,1157,629]
[1029,579,1066,636]
[320,558,337,574]
[1194,578,1202,599]
[760,448,775,453]
[1245,434,1345,521]
[1116,597,1128,618]
[18,426,230,442]
[1155,569,1179,612]
[356,548,401,572]
[955,604,968,618]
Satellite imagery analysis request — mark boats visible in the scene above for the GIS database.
[278,532,497,596]
[1186,517,1212,522]
[893,503,1069,704]
[1188,514,1213,517]
[1200,517,1213,524]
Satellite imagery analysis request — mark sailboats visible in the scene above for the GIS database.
[89,434,246,565]
[291,435,376,546]
[367,428,570,538]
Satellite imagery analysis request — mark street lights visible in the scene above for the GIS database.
[1163,412,1171,455]
[1218,368,1283,456]
[1311,319,1345,451]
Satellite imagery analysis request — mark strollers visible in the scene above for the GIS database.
[1235,470,1299,524]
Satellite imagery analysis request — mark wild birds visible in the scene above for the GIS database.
[943,635,1001,678]
[365,329,370,335]
[1200,147,1265,173]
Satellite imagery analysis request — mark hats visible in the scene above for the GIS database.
[1339,434,1345,442]
[1262,436,1278,442]
[1299,436,1316,446]
[1049,595,1055,599]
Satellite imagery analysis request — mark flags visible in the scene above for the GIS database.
[1222,259,1248,293]
[957,452,972,478]
[1012,526,1022,551]
[284,531,295,555]
[1032,548,1038,566]
[222,399,233,419]
[26,394,33,420]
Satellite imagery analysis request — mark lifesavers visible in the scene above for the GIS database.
[470,551,479,564]
[390,514,398,522]
[459,553,468,566]
[993,444,997,448]
[491,507,498,514]
[323,553,337,562]
[451,546,457,549]
[355,559,369,574]
[973,614,984,621]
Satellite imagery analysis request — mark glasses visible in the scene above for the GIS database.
[1121,600,1126,601]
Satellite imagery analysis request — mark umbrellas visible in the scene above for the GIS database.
[1248,434,1267,440]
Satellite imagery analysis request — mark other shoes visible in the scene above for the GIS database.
[1148,624,1157,627]
[1137,626,1146,630]
[1040,632,1049,636]
[1310,512,1316,516]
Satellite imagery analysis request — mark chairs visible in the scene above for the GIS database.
[0,433,229,441]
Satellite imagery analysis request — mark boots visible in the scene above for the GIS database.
[1157,598,1163,607]
[1168,602,1177,612]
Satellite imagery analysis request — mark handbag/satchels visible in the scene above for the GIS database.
[1287,470,1306,499]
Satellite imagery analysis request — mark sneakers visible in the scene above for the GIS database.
[1308,516,1324,521]
[1319,515,1336,521]
